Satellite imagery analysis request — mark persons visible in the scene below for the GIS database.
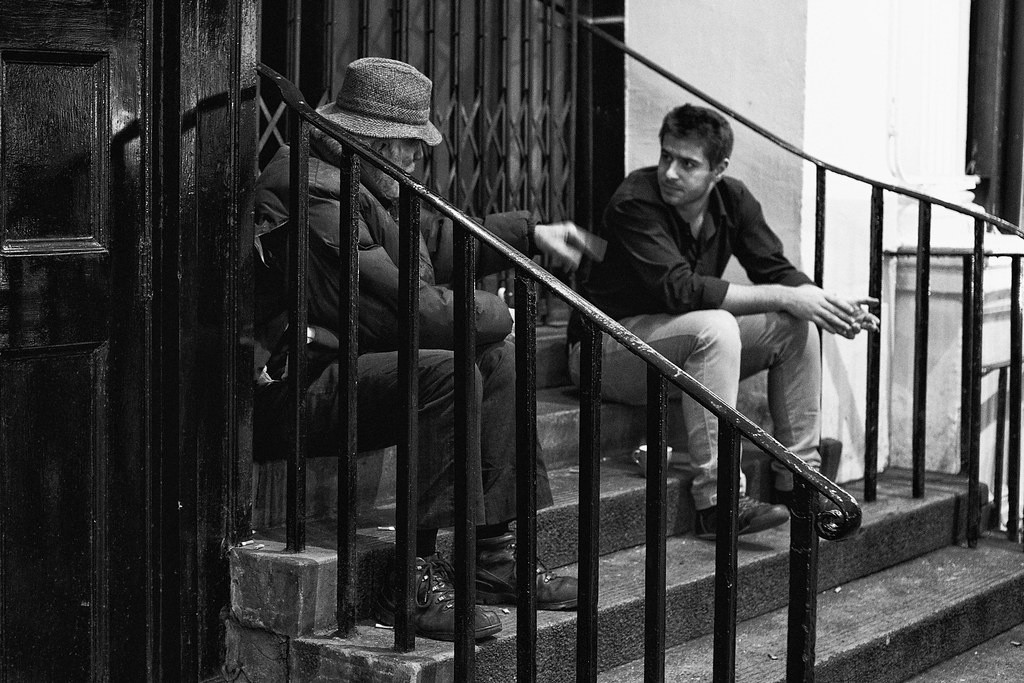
[568,103,880,540]
[256,59,608,640]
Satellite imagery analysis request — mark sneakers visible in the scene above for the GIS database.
[376,552,503,641]
[476,534,578,610]
[773,488,841,513]
[696,492,789,540]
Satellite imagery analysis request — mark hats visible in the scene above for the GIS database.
[314,57,443,146]
